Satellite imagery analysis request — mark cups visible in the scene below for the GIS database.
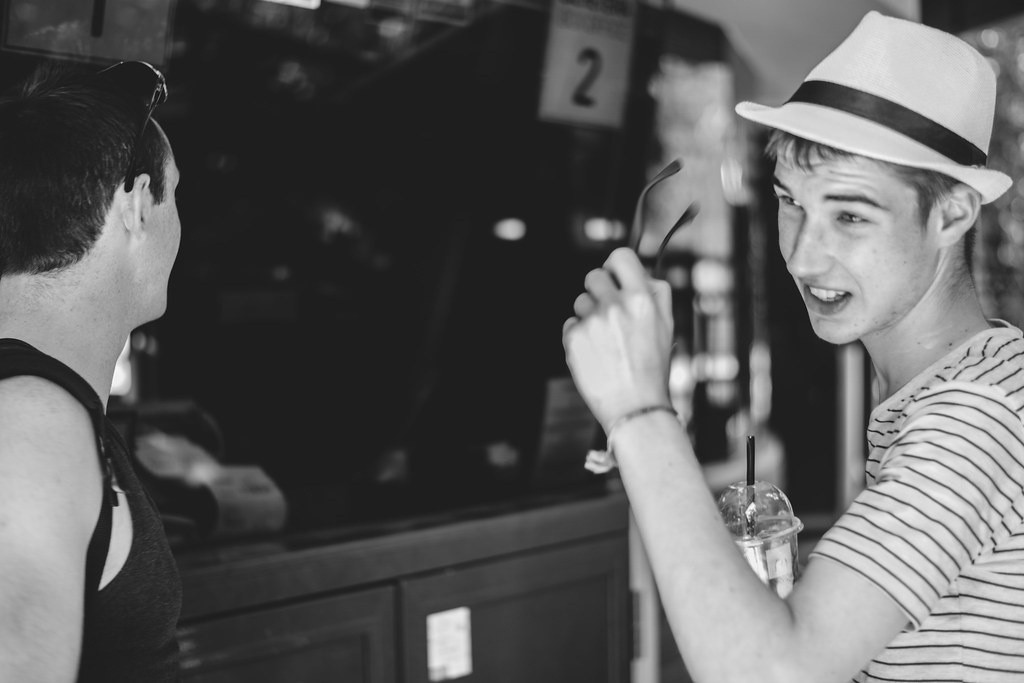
[716,478,803,601]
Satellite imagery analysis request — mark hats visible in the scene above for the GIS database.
[735,10,1014,205]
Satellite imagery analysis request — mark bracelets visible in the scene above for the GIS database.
[583,404,679,474]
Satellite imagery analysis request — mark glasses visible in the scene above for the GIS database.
[84,60,169,192]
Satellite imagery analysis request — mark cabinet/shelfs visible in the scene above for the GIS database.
[170,530,632,683]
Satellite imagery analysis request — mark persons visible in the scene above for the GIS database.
[0,60,283,683]
[562,11,1024,683]
